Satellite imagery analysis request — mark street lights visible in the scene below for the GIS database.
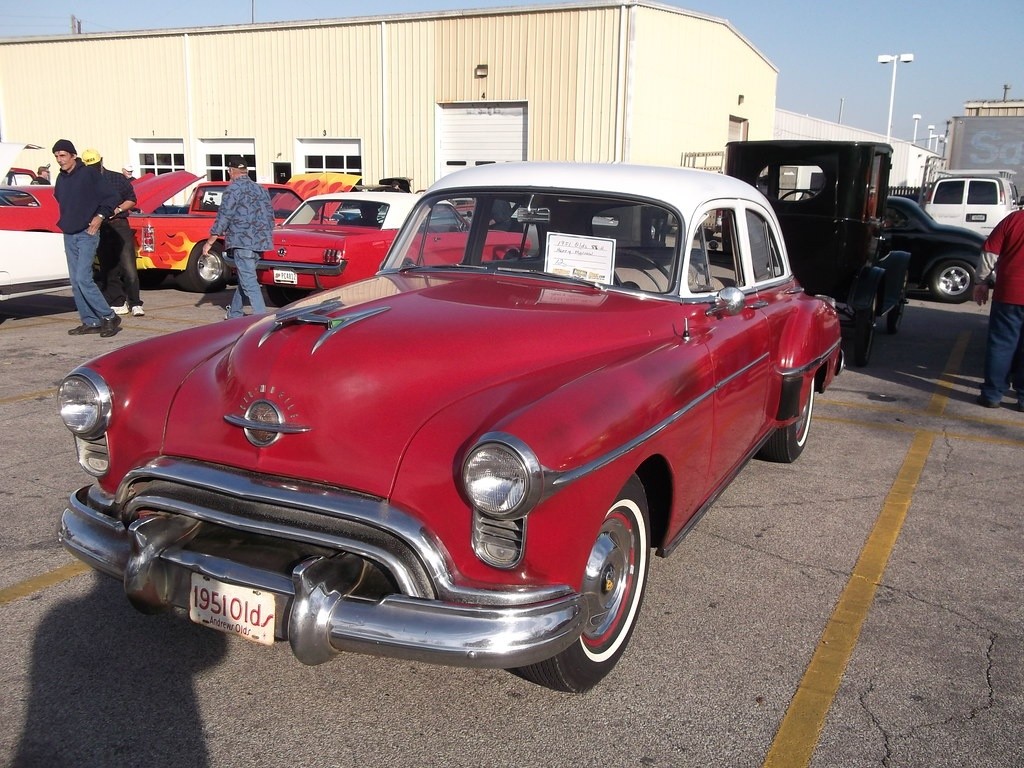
[928,125,935,149]
[913,114,921,144]
[878,53,914,143]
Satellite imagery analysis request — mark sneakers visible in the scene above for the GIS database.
[131,305,145,316]
[110,301,129,314]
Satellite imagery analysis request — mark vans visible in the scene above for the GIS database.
[924,166,1024,239]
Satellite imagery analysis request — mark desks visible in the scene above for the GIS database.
[618,254,737,291]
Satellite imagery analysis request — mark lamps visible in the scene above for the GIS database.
[477,64,488,75]
[738,94,744,104]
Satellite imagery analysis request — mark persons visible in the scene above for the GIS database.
[489,199,512,231]
[81,149,144,316]
[384,181,400,192]
[30,166,51,185]
[972,208,1024,412]
[338,204,380,226]
[52,139,121,336]
[203,156,275,319]
[122,165,136,182]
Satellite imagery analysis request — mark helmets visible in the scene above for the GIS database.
[82,148,101,166]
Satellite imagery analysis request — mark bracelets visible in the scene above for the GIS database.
[118,208,122,212]
[206,241,214,246]
[98,214,105,219]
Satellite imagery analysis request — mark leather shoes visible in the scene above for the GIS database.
[68,323,101,335]
[100,313,122,337]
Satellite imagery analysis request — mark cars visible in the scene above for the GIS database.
[57,161,845,693]
[0,143,530,302]
[882,197,996,303]
[723,140,911,365]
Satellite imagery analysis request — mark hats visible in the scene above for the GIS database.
[226,156,247,168]
[122,163,134,171]
[52,139,76,154]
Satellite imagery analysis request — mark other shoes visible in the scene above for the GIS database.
[1012,403,1024,412]
[977,395,1000,408]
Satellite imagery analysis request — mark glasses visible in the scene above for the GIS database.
[44,171,49,173]
